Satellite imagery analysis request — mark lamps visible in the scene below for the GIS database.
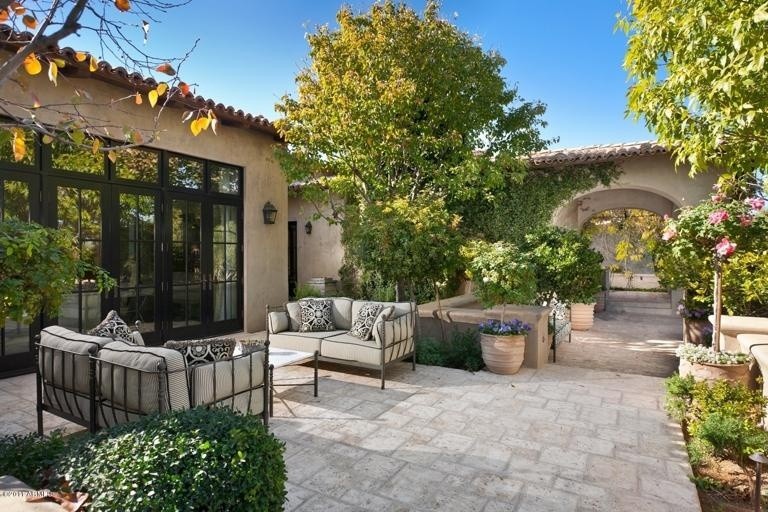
[305,221,312,234]
[262,200,277,225]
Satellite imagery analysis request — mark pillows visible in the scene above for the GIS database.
[86,310,138,346]
[348,303,396,341]
[164,338,237,366]
[299,298,337,333]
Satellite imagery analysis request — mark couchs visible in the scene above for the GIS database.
[266,296,417,390]
[35,325,273,439]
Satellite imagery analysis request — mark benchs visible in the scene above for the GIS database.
[547,303,572,363]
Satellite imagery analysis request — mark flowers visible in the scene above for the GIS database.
[675,340,752,364]
[478,318,532,335]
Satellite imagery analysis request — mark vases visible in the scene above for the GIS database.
[480,333,526,375]
[689,360,750,388]
